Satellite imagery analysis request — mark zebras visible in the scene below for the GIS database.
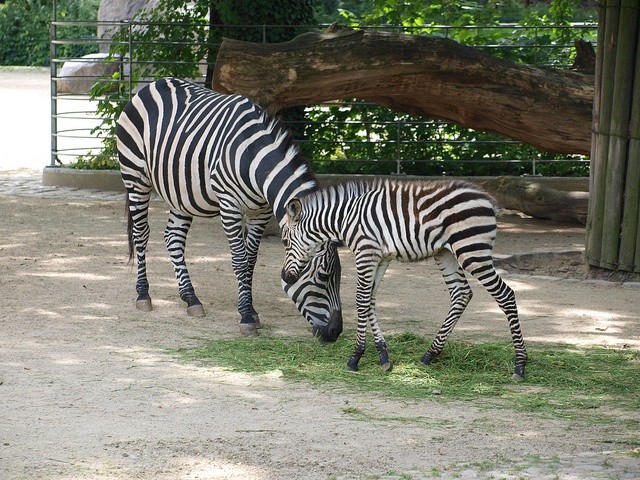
[117,73,344,343]
[277,173,528,384]
